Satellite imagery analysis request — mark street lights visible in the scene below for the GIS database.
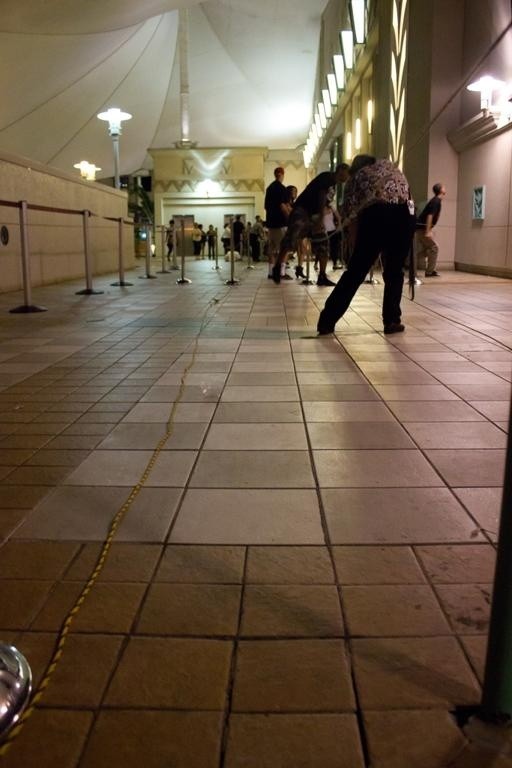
[95,108,134,190]
[86,162,103,180]
[75,158,94,179]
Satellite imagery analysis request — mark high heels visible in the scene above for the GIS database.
[294,266,309,279]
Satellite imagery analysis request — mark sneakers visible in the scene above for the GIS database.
[279,274,293,280]
[316,275,337,286]
[271,266,281,285]
[383,321,406,334]
[424,270,441,277]
[268,274,273,279]
[316,324,335,333]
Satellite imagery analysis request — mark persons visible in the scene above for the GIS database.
[316,154,417,336]
[165,218,180,261]
[416,183,447,277]
[264,162,382,285]
[193,213,266,262]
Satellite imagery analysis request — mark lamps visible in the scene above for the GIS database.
[468,74,512,118]
[73,161,102,182]
[302,0,365,168]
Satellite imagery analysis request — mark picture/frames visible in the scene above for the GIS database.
[471,184,485,220]
